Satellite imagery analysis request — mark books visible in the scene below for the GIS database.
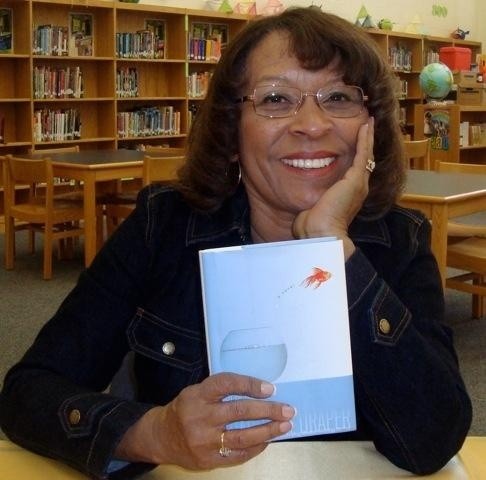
[32,22,222,152]
[388,46,486,148]
[198,236,357,443]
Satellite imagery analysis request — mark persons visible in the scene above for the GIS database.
[0,3,473,480]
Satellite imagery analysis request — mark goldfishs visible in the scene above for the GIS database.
[299,267,332,291]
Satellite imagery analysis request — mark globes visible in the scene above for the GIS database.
[419,62,454,104]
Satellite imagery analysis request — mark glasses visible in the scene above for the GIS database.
[232,84,371,119]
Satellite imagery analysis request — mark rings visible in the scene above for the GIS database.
[365,159,376,172]
[219,431,232,458]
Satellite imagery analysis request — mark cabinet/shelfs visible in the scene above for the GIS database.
[370,30,486,164]
[1,0,227,151]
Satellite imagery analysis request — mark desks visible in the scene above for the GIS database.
[0,435,486,480]
[42,151,152,270]
[394,170,486,301]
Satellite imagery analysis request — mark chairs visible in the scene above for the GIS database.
[405,139,431,171]
[5,154,86,279]
[446,235,486,318]
[107,155,187,242]
